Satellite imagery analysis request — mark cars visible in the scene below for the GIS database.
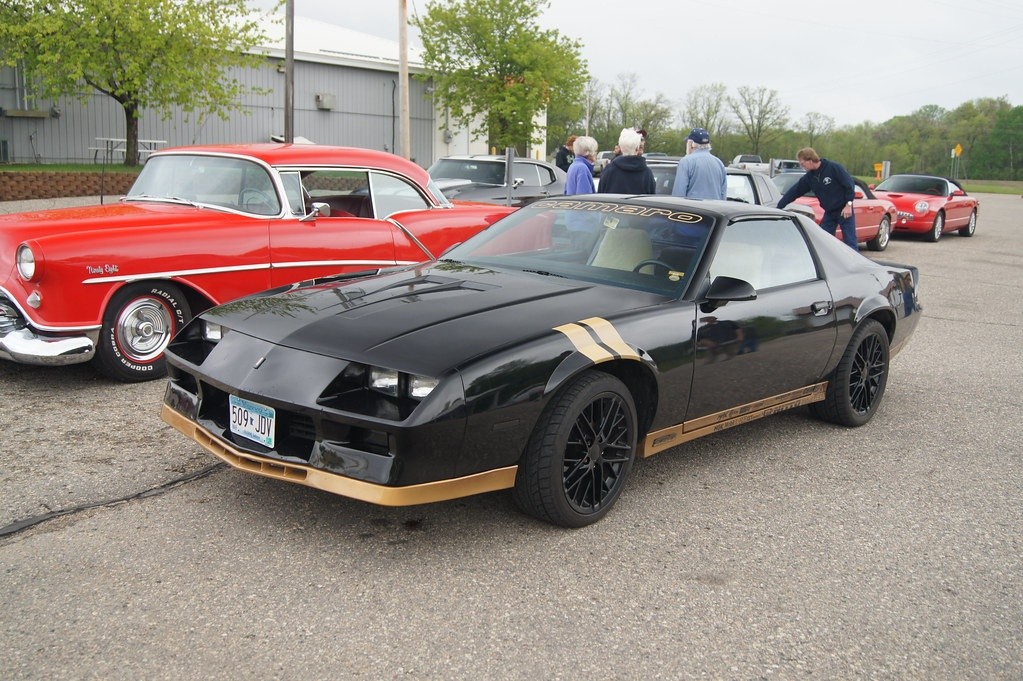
[0,136,558,385]
[426,150,568,208]
[591,150,616,177]
[641,152,817,221]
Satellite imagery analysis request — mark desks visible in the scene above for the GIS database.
[95,137,168,166]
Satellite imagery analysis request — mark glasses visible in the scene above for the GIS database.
[641,138,646,143]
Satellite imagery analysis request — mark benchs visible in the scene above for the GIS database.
[88,146,158,154]
[308,193,372,219]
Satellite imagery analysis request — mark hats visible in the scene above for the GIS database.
[683,128,710,144]
[634,128,647,137]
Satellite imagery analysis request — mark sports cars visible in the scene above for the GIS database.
[772,171,899,252]
[154,193,924,530]
[867,173,981,242]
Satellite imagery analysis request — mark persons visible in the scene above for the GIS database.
[555,127,656,247]
[671,128,728,244]
[775,147,861,253]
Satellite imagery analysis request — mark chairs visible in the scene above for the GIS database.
[589,226,652,274]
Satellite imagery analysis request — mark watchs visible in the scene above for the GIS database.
[847,201,853,205]
